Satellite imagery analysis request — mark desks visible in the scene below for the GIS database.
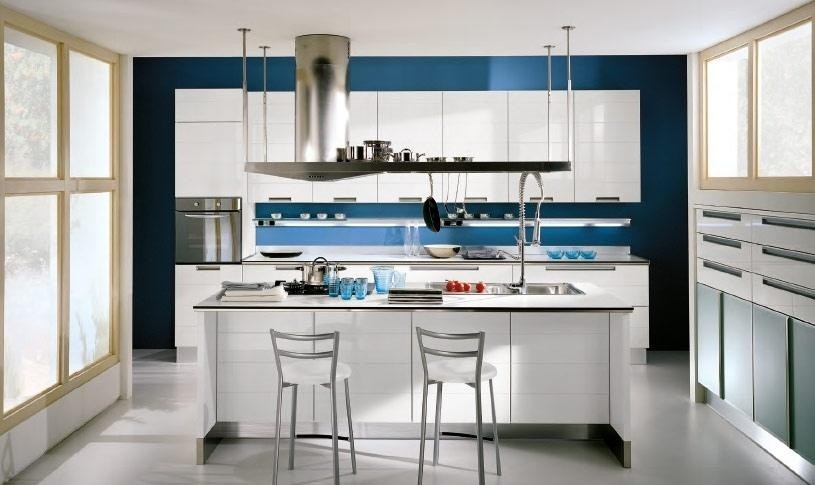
[190,282,638,470]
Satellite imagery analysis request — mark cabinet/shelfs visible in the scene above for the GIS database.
[170,88,652,471]
[752,209,815,466]
[696,205,754,422]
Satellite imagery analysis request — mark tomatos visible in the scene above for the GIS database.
[446,281,486,291]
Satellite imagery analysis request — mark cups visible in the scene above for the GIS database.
[391,270,406,289]
[329,276,369,300]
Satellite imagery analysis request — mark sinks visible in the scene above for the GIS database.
[425,282,504,294]
[525,282,585,295]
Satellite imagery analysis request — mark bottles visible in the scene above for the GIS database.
[403,219,422,257]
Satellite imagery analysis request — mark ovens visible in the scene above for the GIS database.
[175,196,242,265]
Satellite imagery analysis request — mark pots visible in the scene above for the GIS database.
[424,171,442,234]
[336,140,425,162]
[296,255,346,286]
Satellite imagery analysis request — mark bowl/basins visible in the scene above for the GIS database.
[427,156,475,163]
[272,212,348,222]
[547,246,597,261]
[423,243,461,259]
[464,212,517,221]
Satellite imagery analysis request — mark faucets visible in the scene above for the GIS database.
[497,170,545,295]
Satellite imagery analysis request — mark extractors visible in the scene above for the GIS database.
[242,33,390,183]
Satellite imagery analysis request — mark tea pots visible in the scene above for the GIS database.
[370,266,393,293]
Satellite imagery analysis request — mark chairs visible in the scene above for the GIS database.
[415,325,502,485]
[270,328,357,485]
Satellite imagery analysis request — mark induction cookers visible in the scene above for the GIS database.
[282,280,357,296]
